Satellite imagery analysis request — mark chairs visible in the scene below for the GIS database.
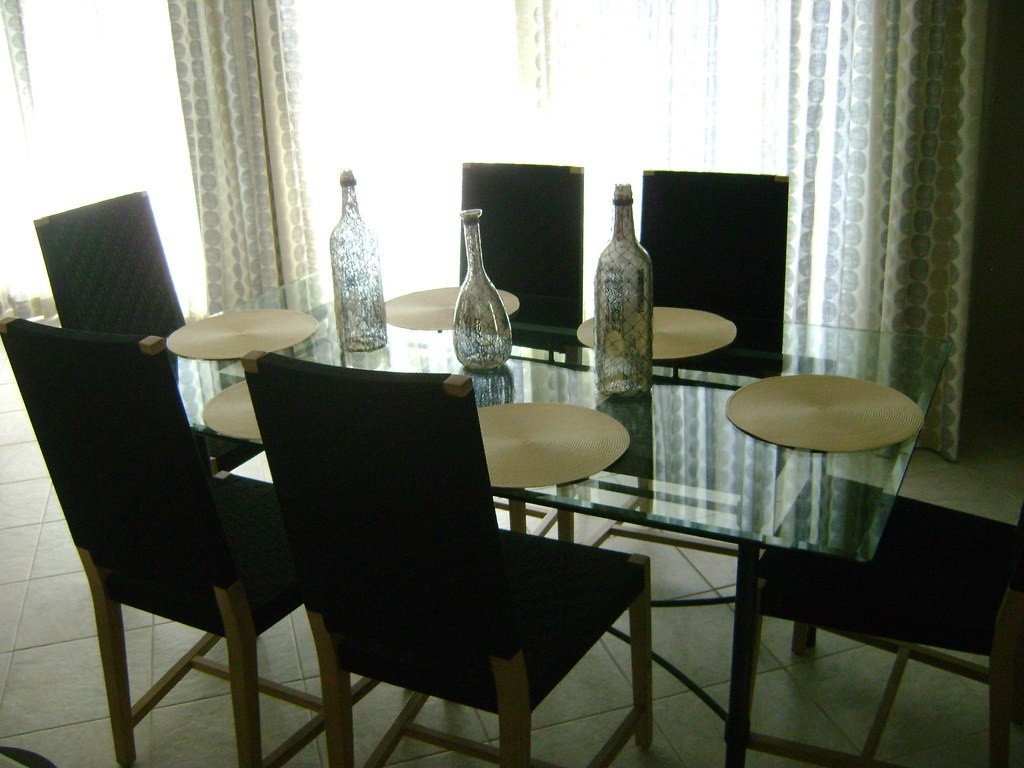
[240,350,655,768]
[582,170,790,548]
[0,317,382,768]
[460,163,587,543]
[35,192,263,474]
[724,473,1024,767]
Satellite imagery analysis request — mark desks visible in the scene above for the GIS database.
[161,270,955,768]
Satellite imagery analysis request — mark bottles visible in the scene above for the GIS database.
[454,209,512,370]
[594,180,654,394]
[331,172,388,352]
